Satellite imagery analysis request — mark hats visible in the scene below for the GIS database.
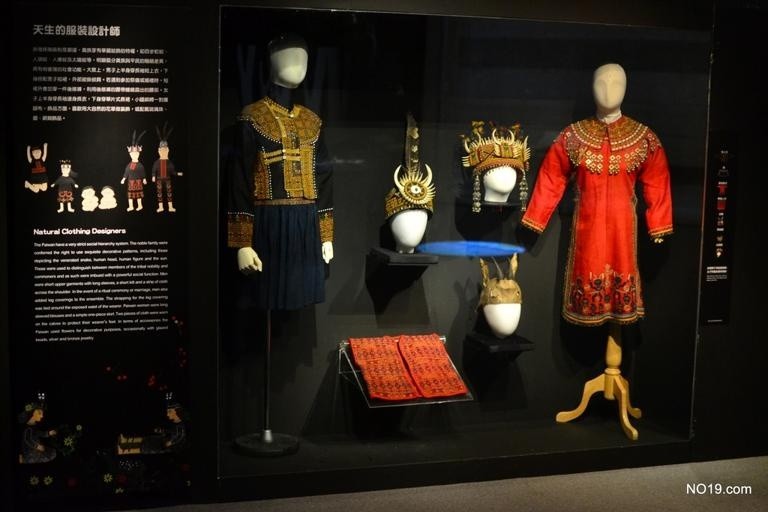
[461,122,530,213]
[476,252,523,307]
[384,164,437,216]
